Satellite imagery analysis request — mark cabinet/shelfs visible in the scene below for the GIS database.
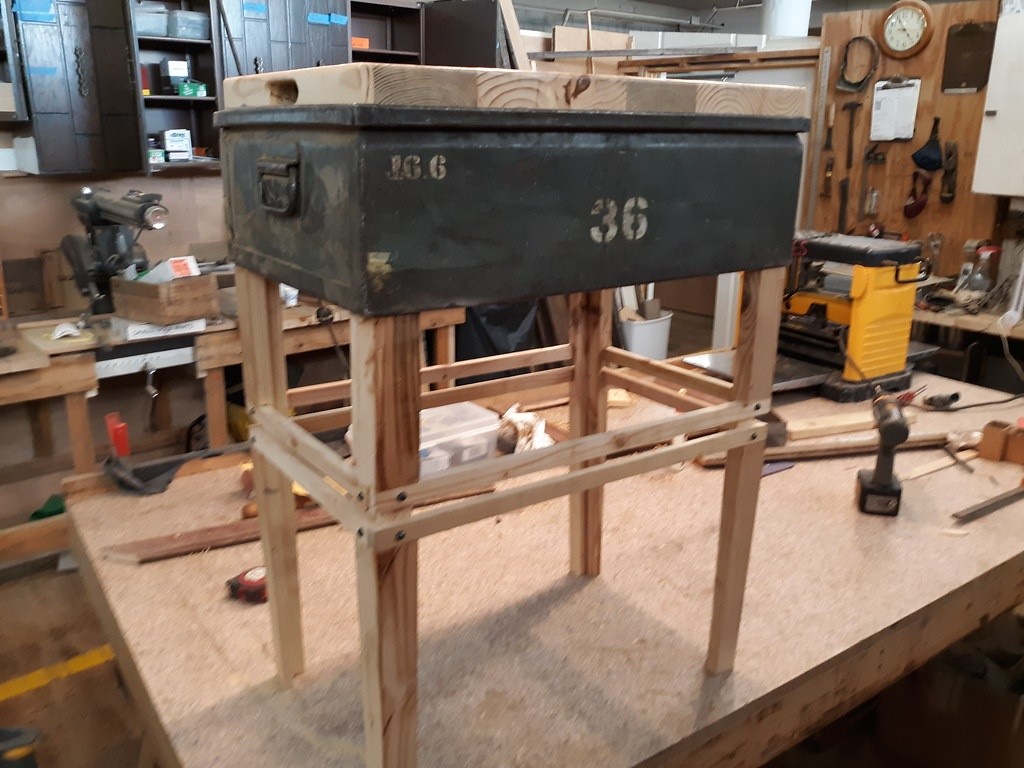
[0,0,427,177]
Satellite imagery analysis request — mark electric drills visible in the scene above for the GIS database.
[856,384,910,517]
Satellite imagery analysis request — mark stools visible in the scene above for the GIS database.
[215,60,806,768]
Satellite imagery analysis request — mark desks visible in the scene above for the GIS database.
[908,268,1023,341]
[2,289,466,566]
[62,337,1023,767]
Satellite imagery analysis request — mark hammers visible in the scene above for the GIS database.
[841,101,860,169]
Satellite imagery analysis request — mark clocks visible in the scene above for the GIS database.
[873,1,934,59]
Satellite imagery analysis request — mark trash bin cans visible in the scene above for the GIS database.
[619,305,674,360]
